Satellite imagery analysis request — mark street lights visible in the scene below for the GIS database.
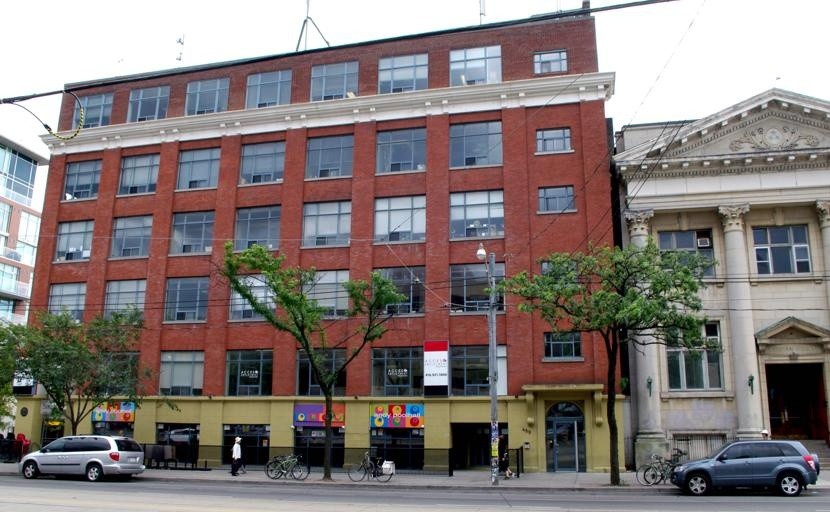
[475,240,500,486]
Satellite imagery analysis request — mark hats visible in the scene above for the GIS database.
[760,430,769,435]
[235,437,242,443]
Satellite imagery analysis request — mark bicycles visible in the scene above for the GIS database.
[264,454,310,482]
[635,448,687,486]
[348,448,394,484]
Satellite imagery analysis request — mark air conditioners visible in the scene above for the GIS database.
[707,337,719,347]
[698,237,710,248]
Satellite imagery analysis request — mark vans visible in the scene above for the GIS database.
[18,434,145,480]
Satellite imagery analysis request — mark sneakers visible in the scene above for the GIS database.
[232,472,238,476]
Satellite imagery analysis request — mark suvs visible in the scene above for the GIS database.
[669,440,821,498]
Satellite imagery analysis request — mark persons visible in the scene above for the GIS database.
[229,437,248,476]
[760,429,770,440]
[502,448,512,481]
